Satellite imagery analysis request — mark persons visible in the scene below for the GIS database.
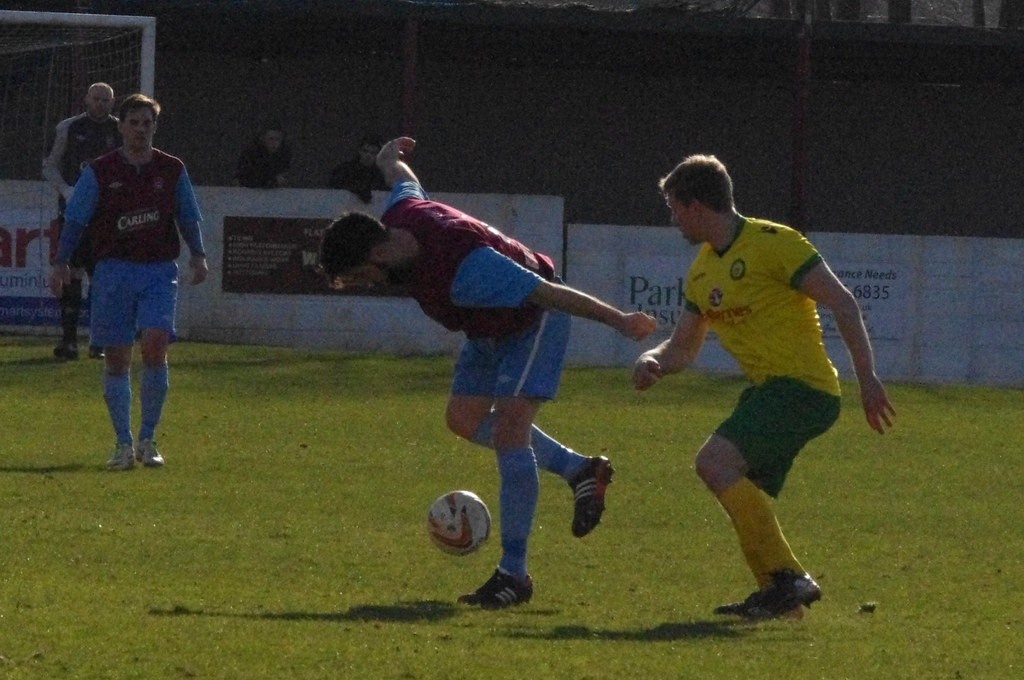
[330,137,386,203]
[629,154,895,619]
[316,137,657,608]
[42,83,123,358]
[237,120,291,188]
[47,96,208,471]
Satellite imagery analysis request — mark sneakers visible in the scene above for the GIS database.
[135,441,164,467]
[456,568,533,610]
[568,455,614,538]
[53,343,80,361]
[713,568,822,622]
[106,445,135,472]
[88,344,105,359]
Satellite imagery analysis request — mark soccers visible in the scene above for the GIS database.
[427,489,493,557]
